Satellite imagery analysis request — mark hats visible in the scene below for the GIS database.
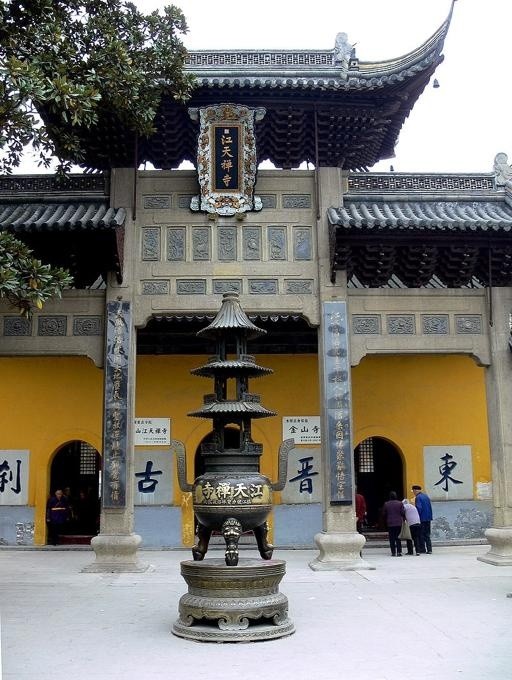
[412,486,421,490]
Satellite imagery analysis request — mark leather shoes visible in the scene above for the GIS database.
[397,550,432,557]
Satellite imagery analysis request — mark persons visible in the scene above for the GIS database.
[355,485,367,557]
[46,488,70,545]
[401,498,421,556]
[411,485,432,554]
[62,486,79,536]
[76,489,90,535]
[381,490,407,557]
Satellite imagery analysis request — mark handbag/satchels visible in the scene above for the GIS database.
[398,520,412,541]
